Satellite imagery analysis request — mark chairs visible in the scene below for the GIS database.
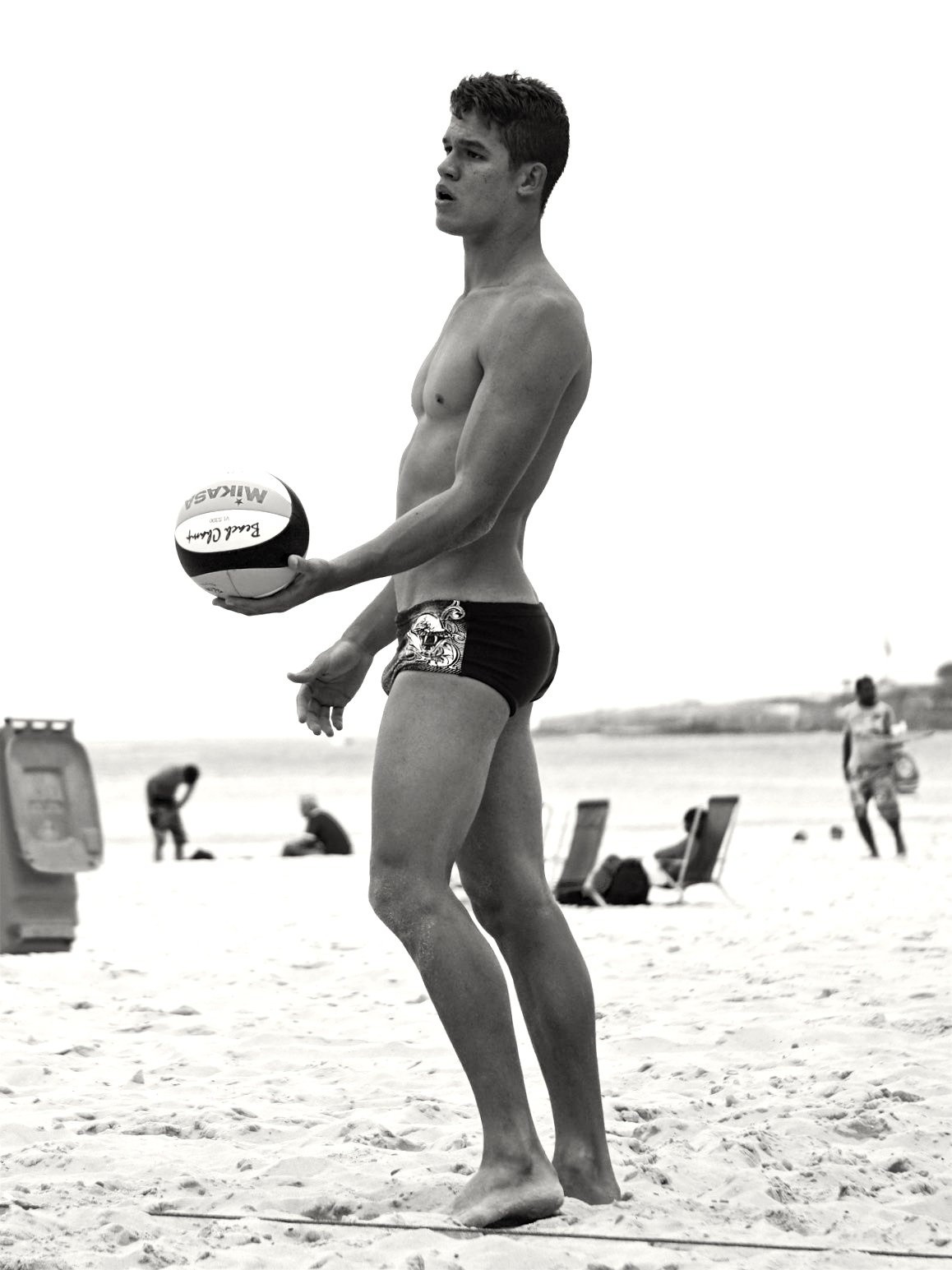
[549,797,611,909]
[639,794,741,906]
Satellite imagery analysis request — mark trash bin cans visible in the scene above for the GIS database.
[1,716,102,954]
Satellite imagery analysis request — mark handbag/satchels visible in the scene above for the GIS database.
[594,853,650,906]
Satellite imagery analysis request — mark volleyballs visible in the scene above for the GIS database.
[175,472,309,599]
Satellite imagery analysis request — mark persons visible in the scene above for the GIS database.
[285,793,351,857]
[210,69,621,1235]
[840,677,907,857]
[655,808,707,890]
[146,766,202,861]
